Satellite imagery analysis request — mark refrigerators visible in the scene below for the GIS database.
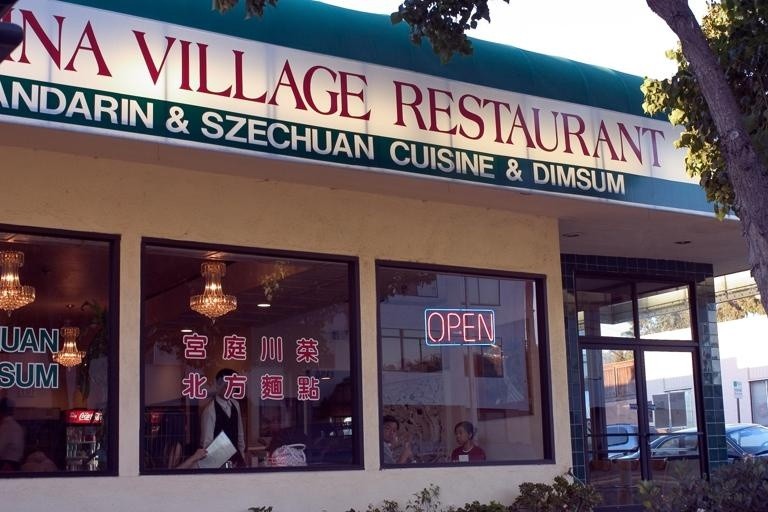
[60,408,108,471]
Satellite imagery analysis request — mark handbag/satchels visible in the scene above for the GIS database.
[271,446,308,467]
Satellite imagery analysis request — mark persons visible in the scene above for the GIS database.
[0,397,26,474]
[379,411,414,467]
[198,367,245,472]
[153,409,208,472]
[451,423,485,464]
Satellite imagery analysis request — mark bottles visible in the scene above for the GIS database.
[65,425,99,471]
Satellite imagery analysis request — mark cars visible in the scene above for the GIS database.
[605,424,767,463]
